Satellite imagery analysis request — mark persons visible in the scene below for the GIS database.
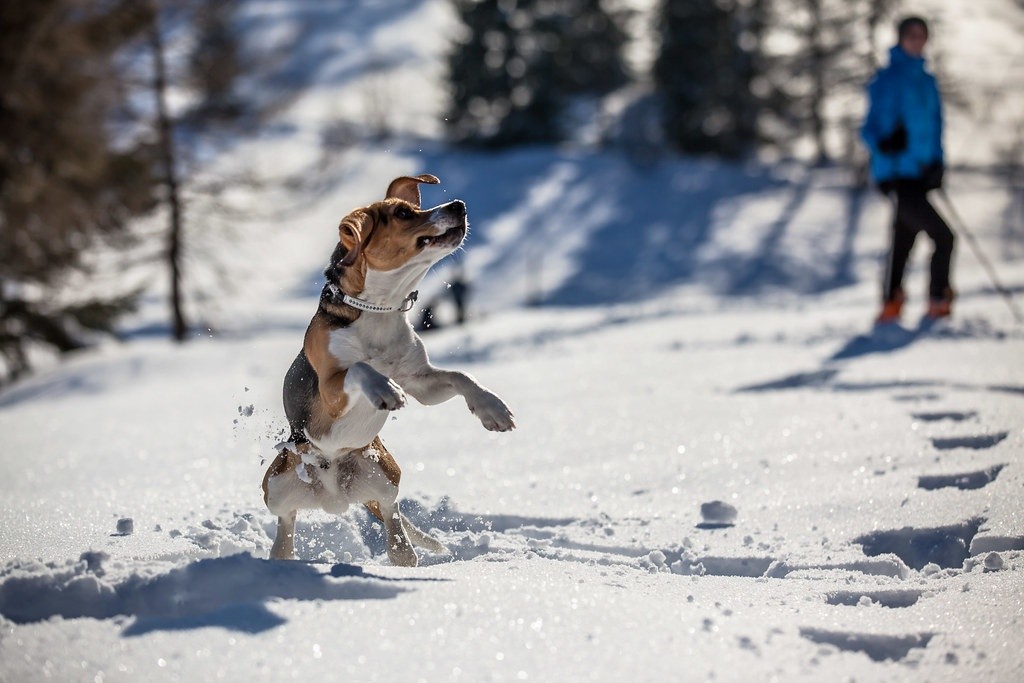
[858,17,955,321]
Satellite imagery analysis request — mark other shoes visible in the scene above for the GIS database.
[880,289,904,321]
[930,290,951,317]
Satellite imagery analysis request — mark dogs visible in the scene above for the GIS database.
[261,171,517,569]
